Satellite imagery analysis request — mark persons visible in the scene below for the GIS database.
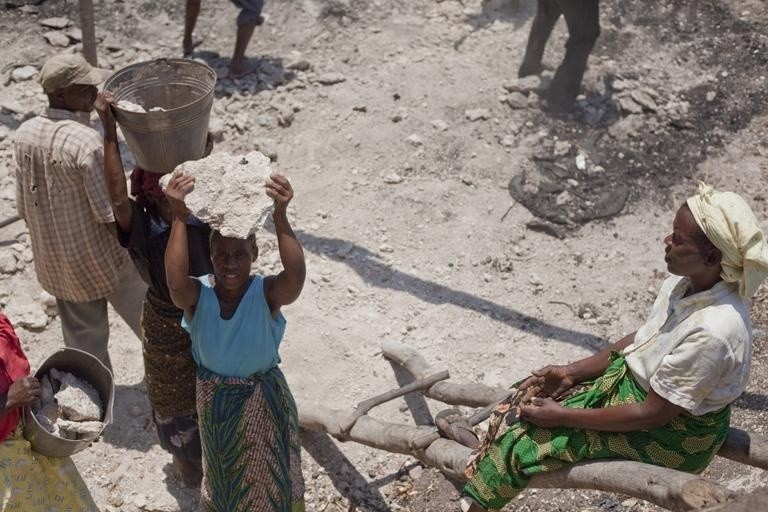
[180,1,268,80]
[0,311,107,511]
[518,1,618,128]
[160,169,309,512]
[434,180,768,508]
[14,54,150,378]
[95,90,216,471]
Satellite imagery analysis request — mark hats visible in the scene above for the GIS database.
[35,53,118,95]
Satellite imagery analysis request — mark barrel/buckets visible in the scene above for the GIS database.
[21,348,115,458]
[103,56,217,174]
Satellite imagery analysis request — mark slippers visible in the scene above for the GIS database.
[433,407,483,450]
